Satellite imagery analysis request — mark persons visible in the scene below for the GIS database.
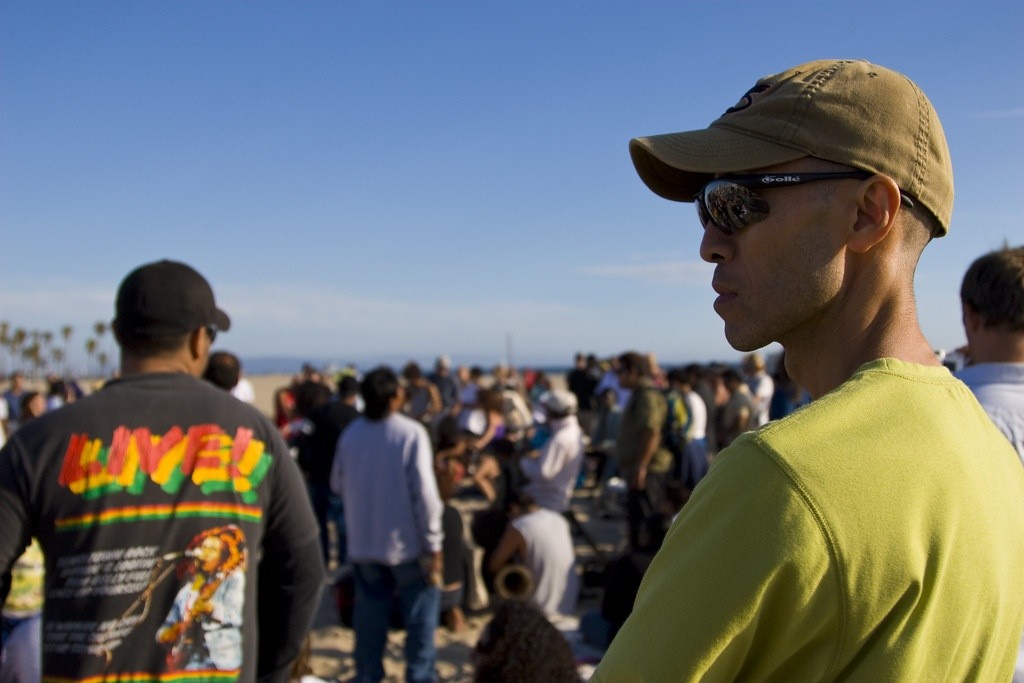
[586,58,1024,683]
[0,246,1024,683]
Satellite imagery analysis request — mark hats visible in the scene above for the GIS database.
[540,388,576,413]
[358,367,394,420]
[115,260,230,332]
[628,56,953,238]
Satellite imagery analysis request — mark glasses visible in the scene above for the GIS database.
[694,171,913,235]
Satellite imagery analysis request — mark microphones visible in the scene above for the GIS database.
[162,548,202,561]
